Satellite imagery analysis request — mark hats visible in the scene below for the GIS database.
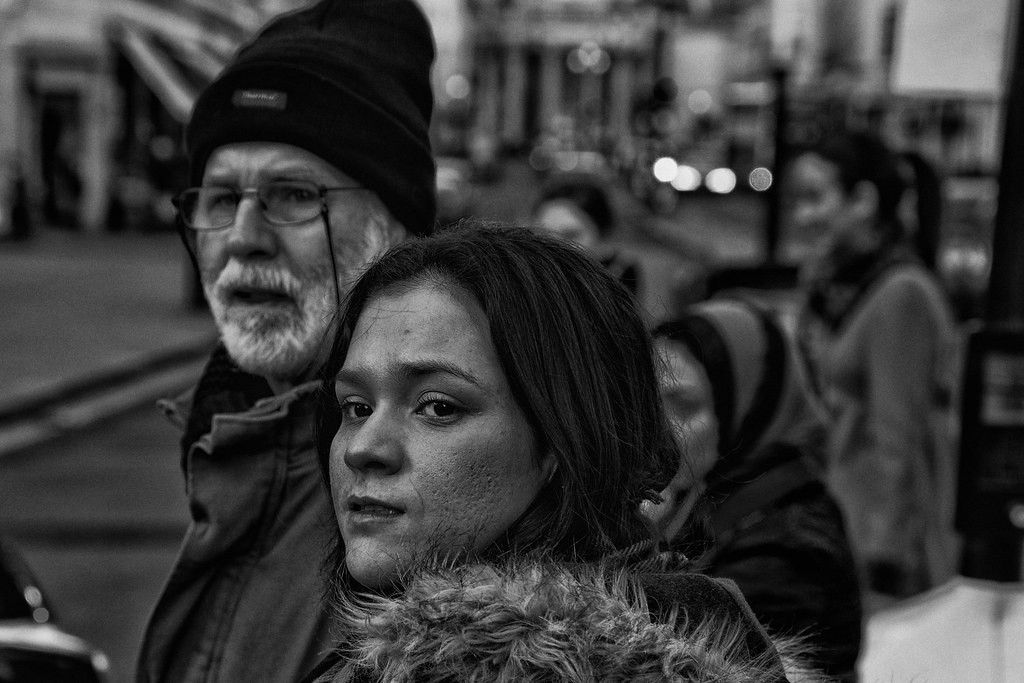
[185,0,439,237]
[652,297,792,483]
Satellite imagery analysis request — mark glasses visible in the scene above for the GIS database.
[172,181,363,229]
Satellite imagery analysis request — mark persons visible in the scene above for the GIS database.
[0,1,983,683]
[137,0,436,682]
